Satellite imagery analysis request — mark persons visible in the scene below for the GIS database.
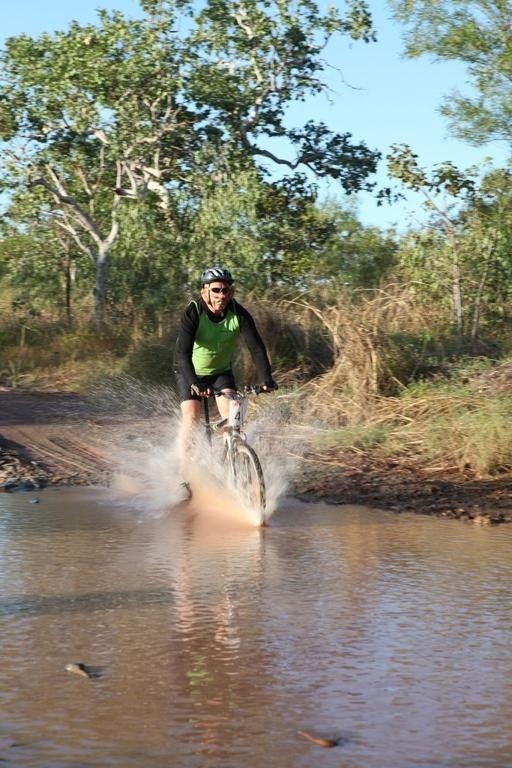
[164,266,283,508]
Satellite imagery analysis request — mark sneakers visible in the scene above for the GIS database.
[178,481,192,502]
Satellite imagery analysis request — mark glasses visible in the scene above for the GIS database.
[209,287,229,293]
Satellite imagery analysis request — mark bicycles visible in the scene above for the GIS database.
[185,375,277,529]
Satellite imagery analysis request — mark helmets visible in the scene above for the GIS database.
[200,268,234,286]
